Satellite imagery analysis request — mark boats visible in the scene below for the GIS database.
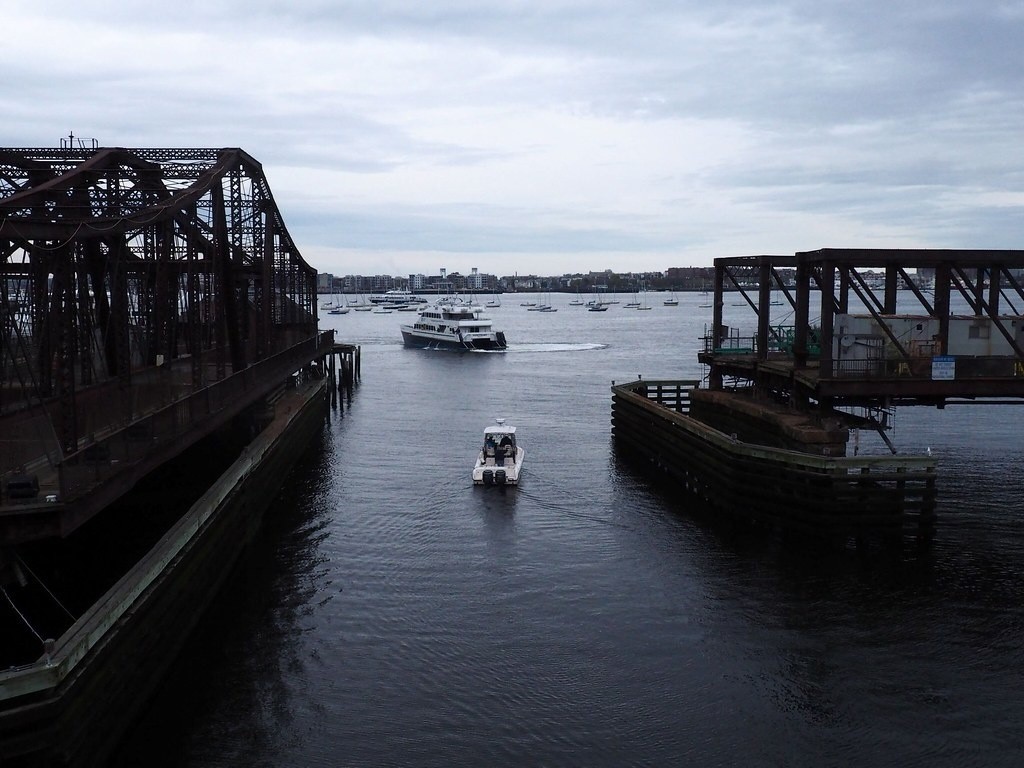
[398,294,508,352]
[471,418,525,484]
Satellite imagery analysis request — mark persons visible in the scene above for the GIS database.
[488,436,498,446]
[500,436,512,447]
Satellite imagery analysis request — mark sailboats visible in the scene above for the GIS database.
[699,289,712,308]
[697,283,708,296]
[320,278,679,314]
[731,291,747,306]
[770,286,784,305]
